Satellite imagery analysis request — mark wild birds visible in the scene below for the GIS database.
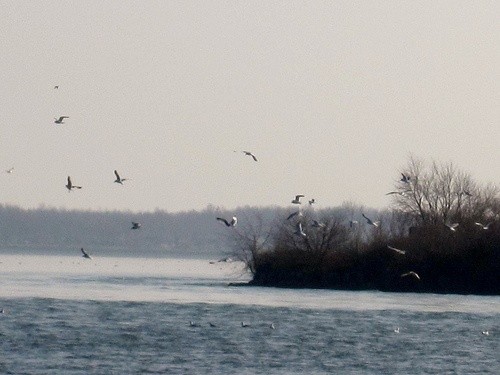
[53,116,69,124]
[481,330,490,336]
[190,320,201,327]
[65,175,82,191]
[445,222,459,231]
[129,221,143,230]
[54,85,59,88]
[81,247,95,257]
[216,216,237,227]
[393,327,400,331]
[271,323,274,329]
[6,167,14,172]
[388,245,406,256]
[113,170,129,184]
[475,221,493,230]
[243,150,257,161]
[209,321,217,327]
[209,257,232,264]
[282,170,473,236]
[241,321,249,325]
